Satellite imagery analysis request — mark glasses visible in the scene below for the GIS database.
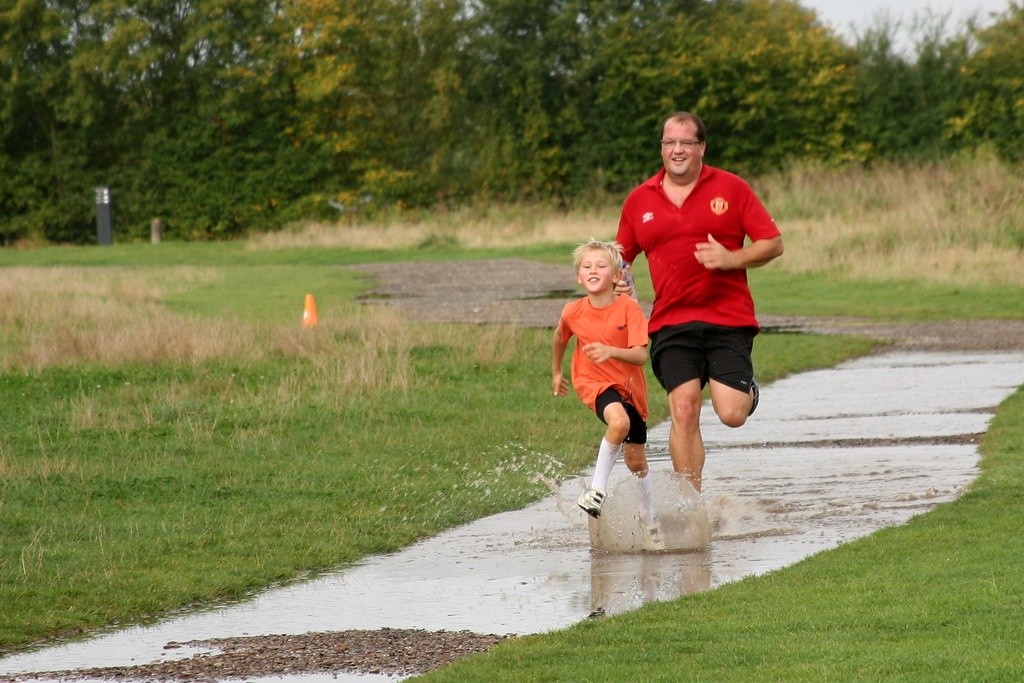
[661,138,702,146]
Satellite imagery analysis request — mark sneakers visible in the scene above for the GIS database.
[749,377,759,416]
[578,487,607,517]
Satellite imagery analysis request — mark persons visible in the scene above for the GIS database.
[611,111,784,512]
[550,234,666,551]
